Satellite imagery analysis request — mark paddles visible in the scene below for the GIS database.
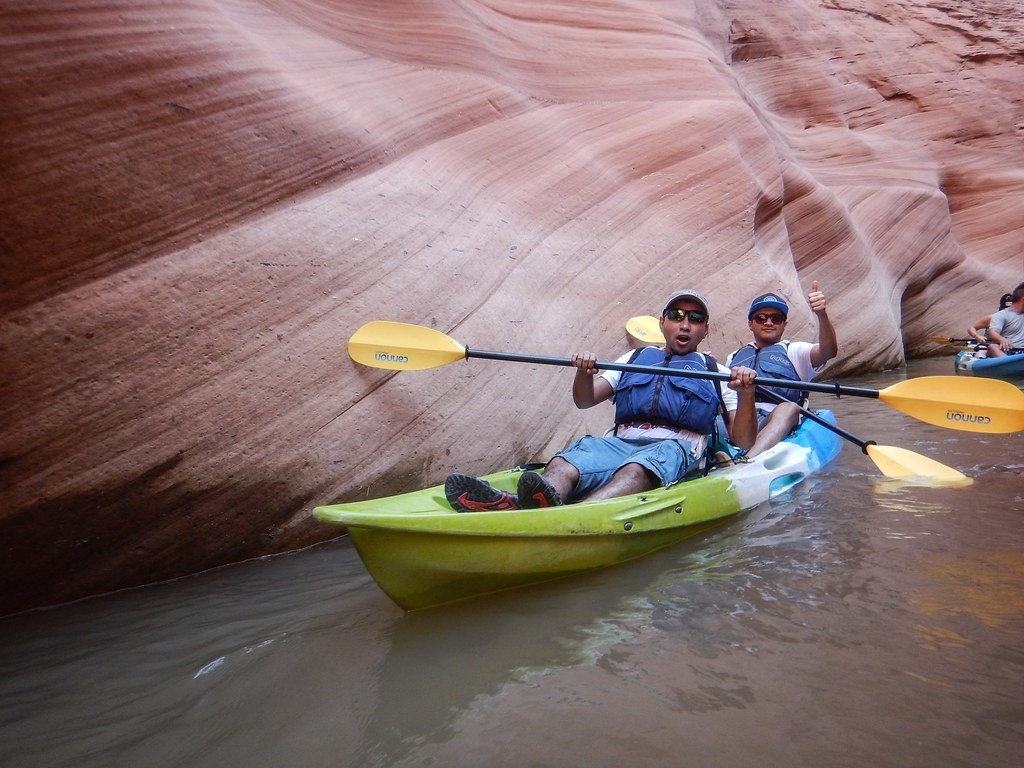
[950,344,987,351]
[928,334,973,345]
[628,314,966,480]
[348,318,1024,435]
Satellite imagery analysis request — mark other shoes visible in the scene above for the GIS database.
[518,471,565,509]
[445,473,524,513]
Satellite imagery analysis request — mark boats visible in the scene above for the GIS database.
[954,350,1024,377]
[312,409,841,613]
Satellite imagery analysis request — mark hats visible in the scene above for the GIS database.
[747,293,788,321]
[661,289,711,322]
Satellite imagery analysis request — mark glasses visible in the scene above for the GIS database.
[664,308,708,324]
[751,313,787,325]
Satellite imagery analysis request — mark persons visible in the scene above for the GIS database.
[967,293,1013,358]
[988,282,1024,358]
[715,280,837,469]
[445,289,758,513]
